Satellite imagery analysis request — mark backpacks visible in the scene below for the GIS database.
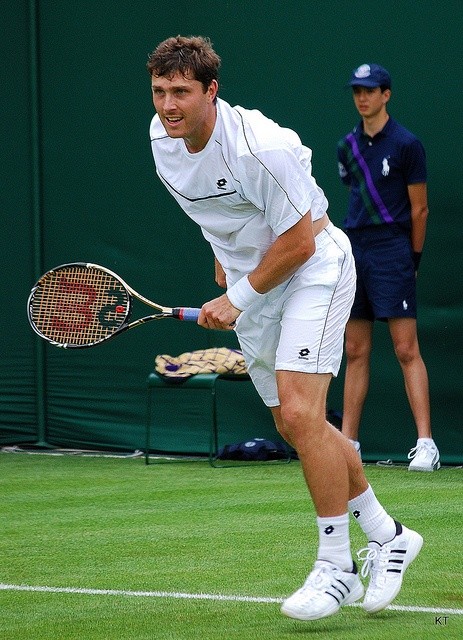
[220,436,284,461]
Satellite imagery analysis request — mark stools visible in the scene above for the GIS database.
[145,372,292,469]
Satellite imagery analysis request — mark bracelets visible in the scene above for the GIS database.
[225,276,263,310]
[411,251,422,273]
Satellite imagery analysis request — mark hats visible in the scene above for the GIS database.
[348,64,391,88]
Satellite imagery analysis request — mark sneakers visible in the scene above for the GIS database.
[408,439,440,472]
[281,559,365,620]
[348,437,361,464]
[357,520,423,614]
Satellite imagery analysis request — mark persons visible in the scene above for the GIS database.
[332,64,441,474]
[145,36,422,620]
